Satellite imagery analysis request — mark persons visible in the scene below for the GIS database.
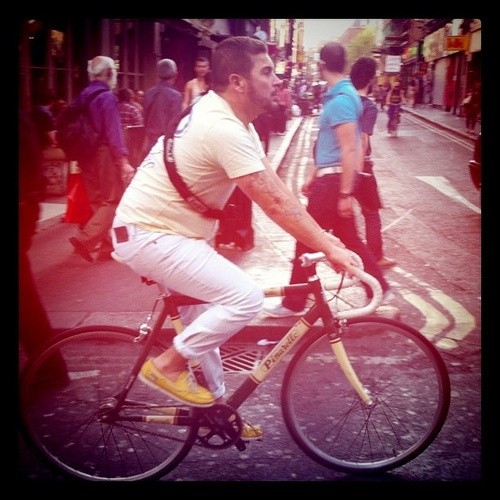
[262,41,392,319]
[386,81,403,134]
[329,57,394,269]
[465,81,479,134]
[379,74,407,111]
[182,57,209,110]
[142,57,182,155]
[19,87,74,396]
[110,38,363,441]
[69,56,134,262]
[252,76,328,153]
[111,88,144,155]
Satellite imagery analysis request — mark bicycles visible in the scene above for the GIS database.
[19,247,453,484]
[388,102,404,137]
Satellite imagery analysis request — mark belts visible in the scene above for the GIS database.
[316,166,342,178]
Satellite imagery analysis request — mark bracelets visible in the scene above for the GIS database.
[338,191,354,199]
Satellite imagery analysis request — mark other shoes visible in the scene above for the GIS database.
[97,254,114,262]
[365,291,394,305]
[376,258,397,269]
[264,302,305,318]
[69,237,94,263]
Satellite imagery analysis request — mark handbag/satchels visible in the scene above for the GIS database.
[64,172,94,223]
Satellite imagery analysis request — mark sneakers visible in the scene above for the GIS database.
[226,423,262,441]
[138,358,214,408]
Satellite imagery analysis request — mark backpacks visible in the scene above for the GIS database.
[55,89,108,160]
